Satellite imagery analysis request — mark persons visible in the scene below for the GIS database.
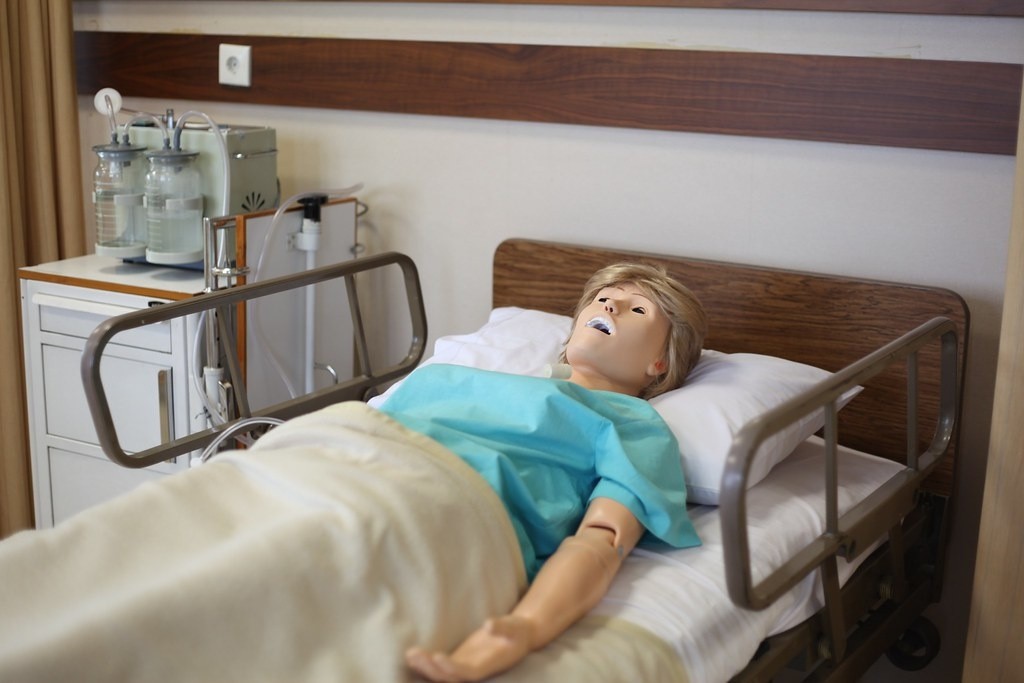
[354,259,707,682]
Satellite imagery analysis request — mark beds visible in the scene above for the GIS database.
[0,237,973,683]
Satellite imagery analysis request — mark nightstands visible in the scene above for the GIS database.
[16,254,239,533]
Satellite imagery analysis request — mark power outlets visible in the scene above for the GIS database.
[218,44,251,87]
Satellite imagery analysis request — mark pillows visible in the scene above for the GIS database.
[366,307,866,507]
